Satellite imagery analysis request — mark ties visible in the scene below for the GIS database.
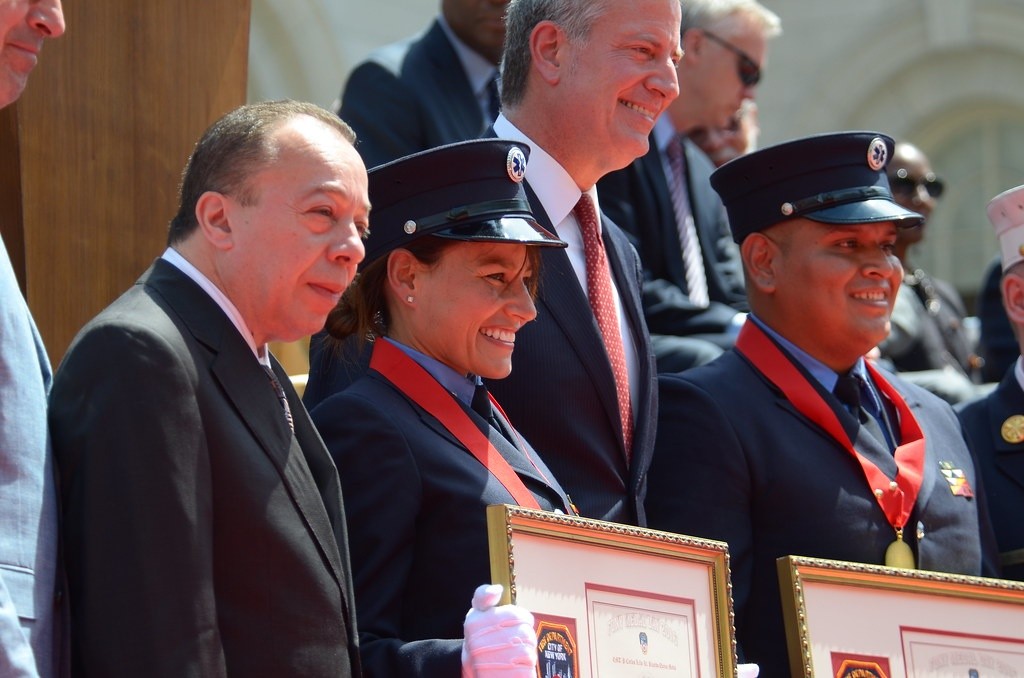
[487,72,501,122]
[572,191,635,470]
[665,134,711,308]
[471,384,503,436]
[260,364,294,434]
[834,372,891,455]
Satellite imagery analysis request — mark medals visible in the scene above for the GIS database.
[885,538,914,570]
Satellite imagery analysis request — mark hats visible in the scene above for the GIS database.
[357,138,569,273]
[708,130,927,245]
[985,184,1024,275]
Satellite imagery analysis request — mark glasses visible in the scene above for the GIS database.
[887,173,945,198]
[689,26,761,86]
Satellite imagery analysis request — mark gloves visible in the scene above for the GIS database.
[460,583,538,678]
[737,664,759,678]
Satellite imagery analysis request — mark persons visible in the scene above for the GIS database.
[323,0,1024,677]
[0,0,66,678]
[39,99,359,677]
